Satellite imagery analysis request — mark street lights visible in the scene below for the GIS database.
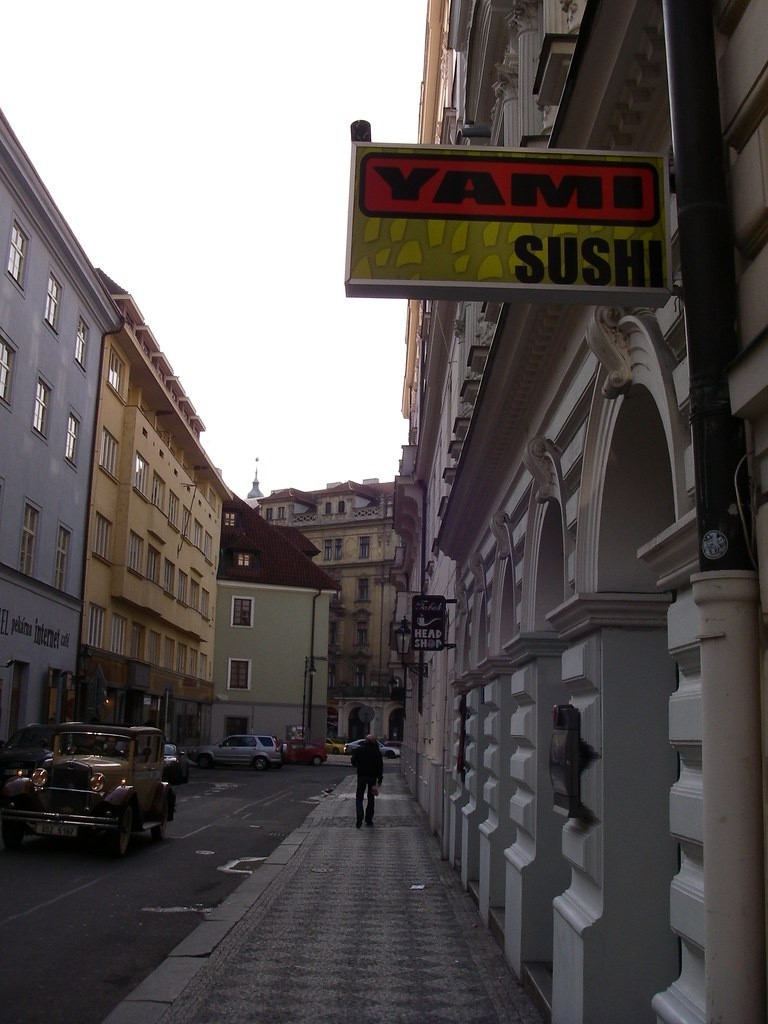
[301,653,317,738]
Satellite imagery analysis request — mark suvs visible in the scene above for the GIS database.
[188,734,283,771]
[0,722,84,783]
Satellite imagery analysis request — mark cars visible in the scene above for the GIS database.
[164,743,186,777]
[344,739,401,759]
[326,737,345,754]
[384,740,401,748]
[278,739,328,766]
[1,723,179,858]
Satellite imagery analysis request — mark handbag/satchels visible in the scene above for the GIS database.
[372,783,380,797]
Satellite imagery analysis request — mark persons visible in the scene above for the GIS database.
[351,735,384,828]
[66,734,128,759]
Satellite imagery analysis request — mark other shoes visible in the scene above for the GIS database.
[365,821,373,825]
[355,822,361,828]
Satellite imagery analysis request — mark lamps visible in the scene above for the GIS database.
[388,671,406,720]
[393,613,429,677]
[0,659,14,667]
[71,643,93,685]
